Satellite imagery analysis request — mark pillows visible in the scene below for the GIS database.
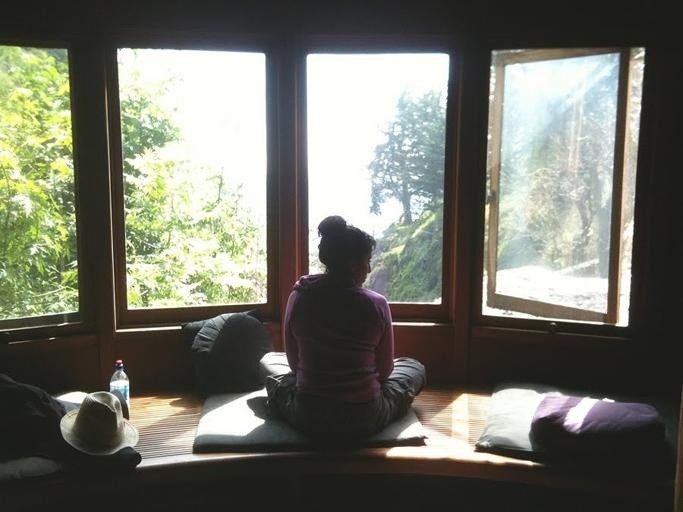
[532,393,665,470]
[180,309,263,398]
[476,380,615,456]
[192,313,274,395]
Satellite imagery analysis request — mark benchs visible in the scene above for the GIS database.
[99,385,683,499]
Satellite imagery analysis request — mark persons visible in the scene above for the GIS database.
[256,214,426,442]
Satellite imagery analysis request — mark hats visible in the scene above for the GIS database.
[57,390,141,458]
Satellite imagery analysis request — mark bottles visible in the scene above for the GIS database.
[110,360,130,420]
